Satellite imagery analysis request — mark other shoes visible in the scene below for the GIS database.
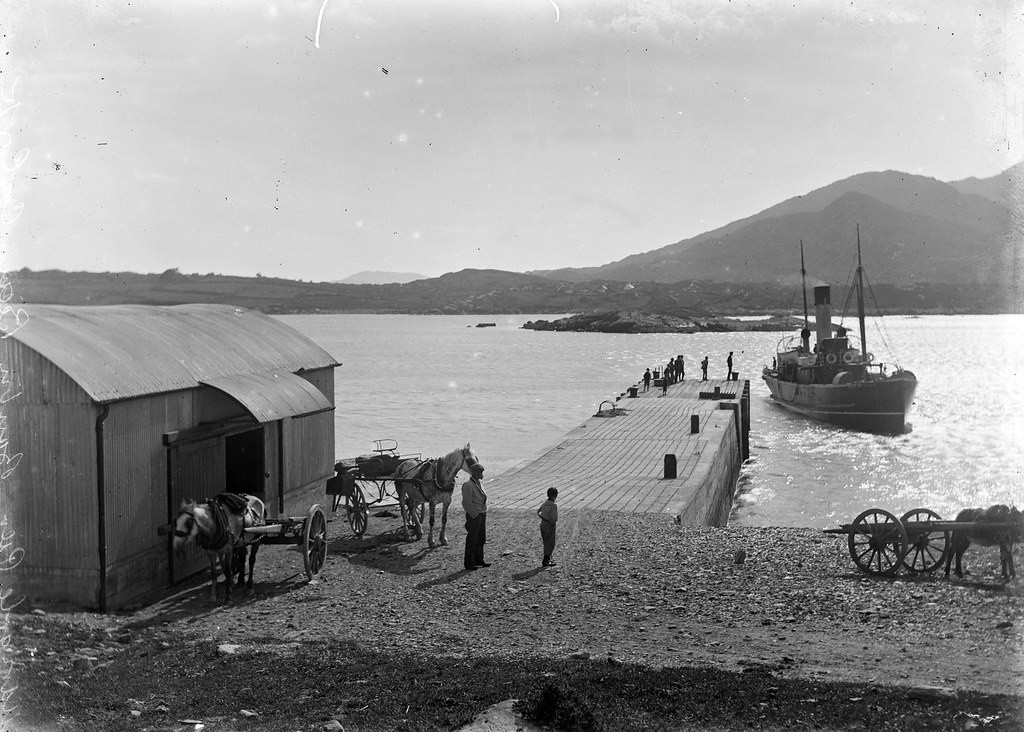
[542,560,557,566]
[475,559,492,567]
[463,565,478,570]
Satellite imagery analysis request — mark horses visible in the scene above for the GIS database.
[394,442,480,549]
[944,505,1024,583]
[172,494,265,604]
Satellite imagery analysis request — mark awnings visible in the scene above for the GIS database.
[200,370,334,426]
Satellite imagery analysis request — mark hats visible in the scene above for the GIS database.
[470,463,485,471]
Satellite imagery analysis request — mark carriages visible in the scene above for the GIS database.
[823,505,1024,582]
[325,438,480,550]
[171,491,329,607]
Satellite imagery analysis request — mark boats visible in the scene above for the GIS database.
[761,222,920,436]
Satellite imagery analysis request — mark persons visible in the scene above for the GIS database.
[537,488,559,567]
[644,368,650,391]
[701,356,709,381]
[727,351,734,380]
[773,356,776,369]
[661,355,685,395]
[462,465,492,570]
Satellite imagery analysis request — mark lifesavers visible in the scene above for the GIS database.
[826,353,837,365]
[842,352,854,364]
